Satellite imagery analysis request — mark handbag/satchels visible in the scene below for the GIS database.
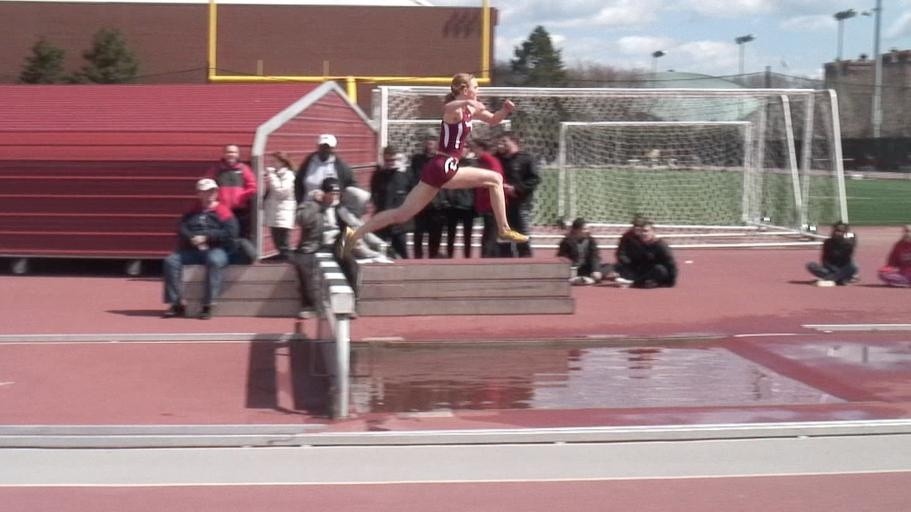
[232,237,256,264]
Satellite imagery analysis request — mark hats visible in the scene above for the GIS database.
[319,134,337,148]
[321,178,342,192]
[196,179,219,191]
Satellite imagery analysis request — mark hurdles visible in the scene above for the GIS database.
[312,253,358,420]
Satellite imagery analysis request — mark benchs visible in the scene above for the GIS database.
[166,253,580,326]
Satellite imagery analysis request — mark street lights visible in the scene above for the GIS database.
[651,49,666,73]
[734,33,758,73]
[834,8,857,60]
[861,0,882,167]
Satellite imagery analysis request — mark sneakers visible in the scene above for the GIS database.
[337,226,357,259]
[497,230,532,244]
[200,306,210,319]
[164,304,187,317]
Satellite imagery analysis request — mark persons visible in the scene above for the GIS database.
[808,220,861,286]
[877,224,911,286]
